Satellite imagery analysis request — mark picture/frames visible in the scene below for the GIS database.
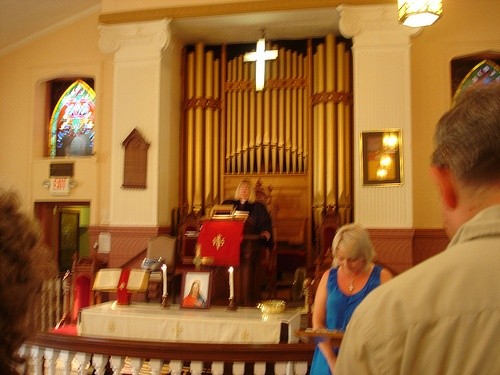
[359,128,405,188]
[178,268,213,311]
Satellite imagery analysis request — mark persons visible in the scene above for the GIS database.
[342,77,500,375]
[0,192,48,375]
[310,224,394,375]
[217,178,274,248]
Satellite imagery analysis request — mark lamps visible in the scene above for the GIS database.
[397,0,443,28]
[42,176,78,196]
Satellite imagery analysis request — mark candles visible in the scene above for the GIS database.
[161,264,167,297]
[228,267,234,298]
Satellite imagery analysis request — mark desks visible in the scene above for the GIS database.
[77,300,301,375]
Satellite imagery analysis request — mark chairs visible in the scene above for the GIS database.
[53,251,109,330]
[253,176,280,300]
[287,203,352,328]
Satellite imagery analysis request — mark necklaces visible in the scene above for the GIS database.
[347,278,354,292]
